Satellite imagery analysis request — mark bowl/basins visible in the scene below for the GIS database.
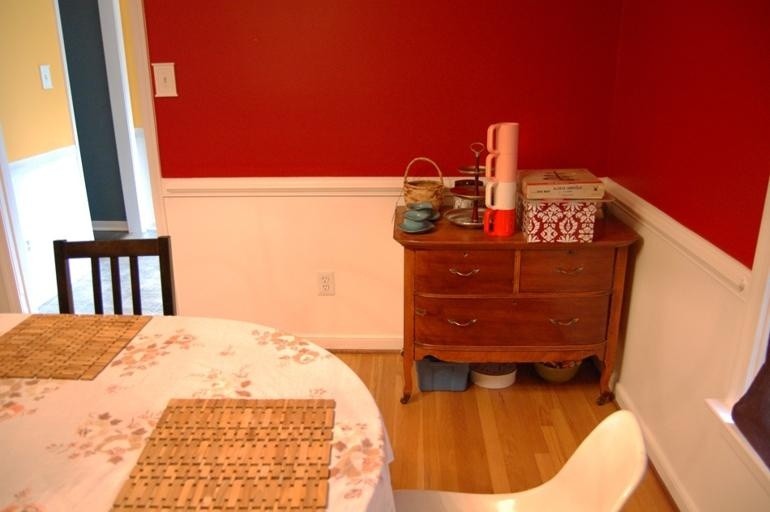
[533,363,581,385]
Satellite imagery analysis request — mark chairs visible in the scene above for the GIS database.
[50,236,177,315]
[391,410,649,512]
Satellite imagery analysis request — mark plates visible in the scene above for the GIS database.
[401,209,441,220]
[398,221,436,234]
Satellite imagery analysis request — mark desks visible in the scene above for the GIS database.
[0,310,389,512]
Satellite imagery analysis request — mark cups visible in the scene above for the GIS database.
[486,122,520,153]
[405,201,434,218]
[402,210,430,229]
[483,209,516,238]
[484,182,516,210]
[484,153,519,181]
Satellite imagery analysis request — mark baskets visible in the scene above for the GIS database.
[403,158,444,210]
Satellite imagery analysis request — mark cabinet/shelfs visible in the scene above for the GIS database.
[395,204,641,405]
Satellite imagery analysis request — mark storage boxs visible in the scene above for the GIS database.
[415,357,470,392]
[518,191,597,243]
